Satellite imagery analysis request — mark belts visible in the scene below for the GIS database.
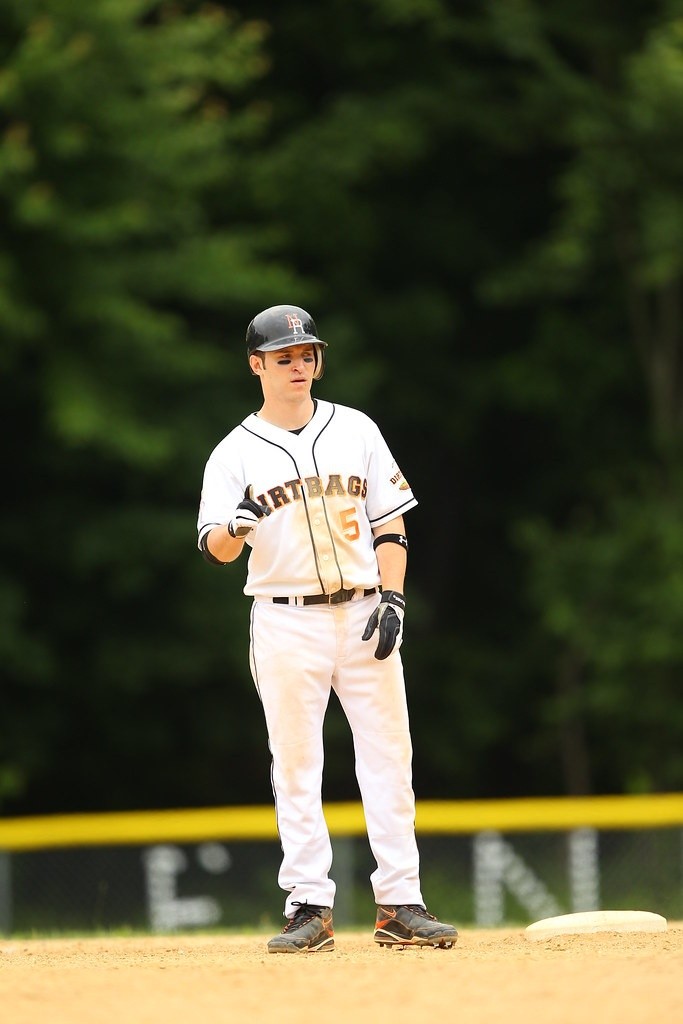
[273,588,376,605]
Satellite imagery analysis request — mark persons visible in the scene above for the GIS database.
[196,305,458,954]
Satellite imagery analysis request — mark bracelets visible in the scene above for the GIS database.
[373,533,408,551]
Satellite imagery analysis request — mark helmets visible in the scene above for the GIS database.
[246,305,328,359]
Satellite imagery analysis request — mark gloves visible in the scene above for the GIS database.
[362,591,406,660]
[227,484,272,539]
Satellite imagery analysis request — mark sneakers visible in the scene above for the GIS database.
[373,904,458,950]
[267,899,335,953]
